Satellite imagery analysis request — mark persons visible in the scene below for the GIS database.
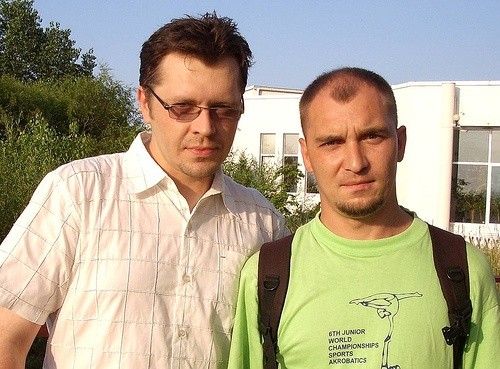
[0,6,291,369]
[228,68,499,369]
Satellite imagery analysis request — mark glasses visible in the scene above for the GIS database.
[144,83,245,121]
